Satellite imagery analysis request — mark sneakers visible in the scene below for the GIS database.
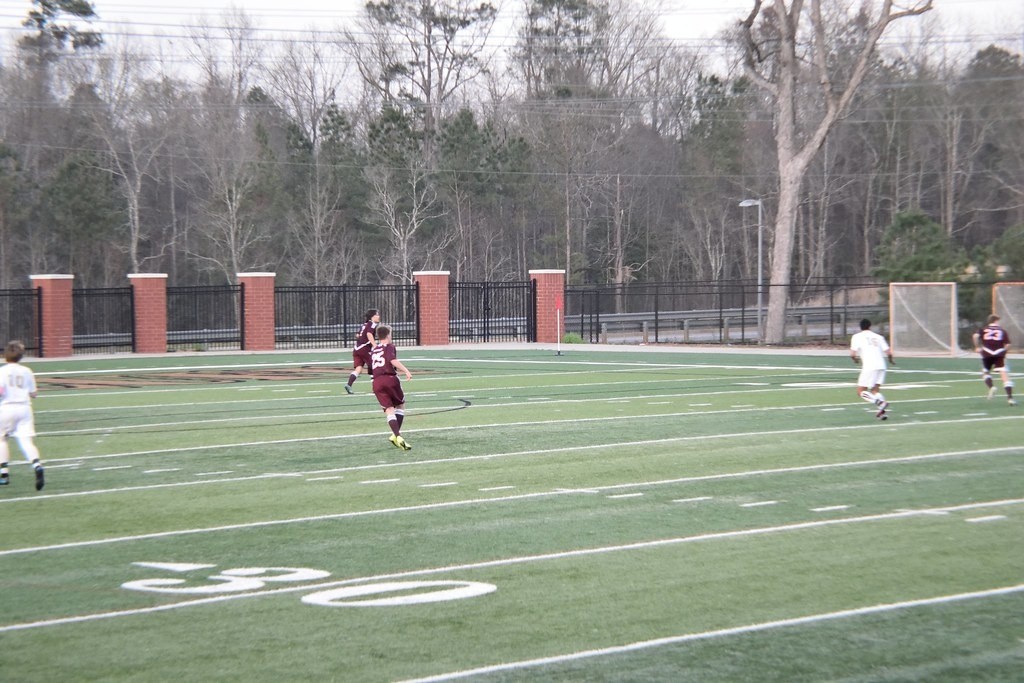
[1008,399,1017,406]
[876,401,888,418]
[988,387,997,399]
[397,436,412,450]
[345,385,354,394]
[389,434,400,448]
[880,412,888,420]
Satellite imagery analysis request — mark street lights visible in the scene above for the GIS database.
[738,198,762,346]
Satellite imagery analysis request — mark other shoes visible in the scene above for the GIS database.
[35,466,44,490]
[0,477,9,486]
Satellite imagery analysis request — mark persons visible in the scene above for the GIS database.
[972,314,1017,406]
[850,319,896,421]
[0,340,45,491]
[344,309,380,395]
[369,323,412,452]
